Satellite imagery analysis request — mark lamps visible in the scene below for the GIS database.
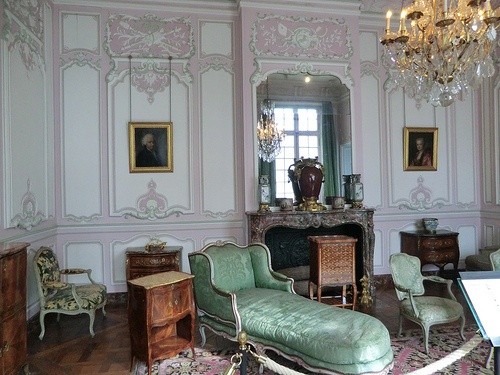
[256,76,285,163]
[378,0,500,112]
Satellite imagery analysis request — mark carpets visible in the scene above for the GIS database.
[133,324,494,375]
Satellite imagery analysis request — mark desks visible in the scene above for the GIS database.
[308,234,358,311]
[400,228,460,288]
[245,207,377,304]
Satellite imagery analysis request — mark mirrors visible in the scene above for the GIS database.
[258,71,353,206]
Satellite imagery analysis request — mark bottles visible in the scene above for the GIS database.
[350,174,364,209]
[294,156,326,211]
[259,175,271,213]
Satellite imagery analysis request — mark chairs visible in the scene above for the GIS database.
[388,252,466,356]
[33,245,107,339]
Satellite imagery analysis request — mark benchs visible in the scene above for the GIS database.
[187,238,395,375]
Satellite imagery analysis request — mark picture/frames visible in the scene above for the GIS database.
[404,126,438,171]
[127,122,174,174]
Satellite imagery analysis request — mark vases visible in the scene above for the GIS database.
[258,174,272,214]
[349,174,364,209]
[341,175,349,203]
[293,156,326,211]
[287,158,303,206]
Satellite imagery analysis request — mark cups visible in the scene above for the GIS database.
[423,218,438,234]
[332,197,345,211]
[281,198,293,211]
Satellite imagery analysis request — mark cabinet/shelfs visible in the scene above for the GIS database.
[127,270,195,375]
[0,241,31,375]
[126,245,182,279]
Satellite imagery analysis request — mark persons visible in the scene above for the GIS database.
[411,137,431,166]
[135,132,163,166]
